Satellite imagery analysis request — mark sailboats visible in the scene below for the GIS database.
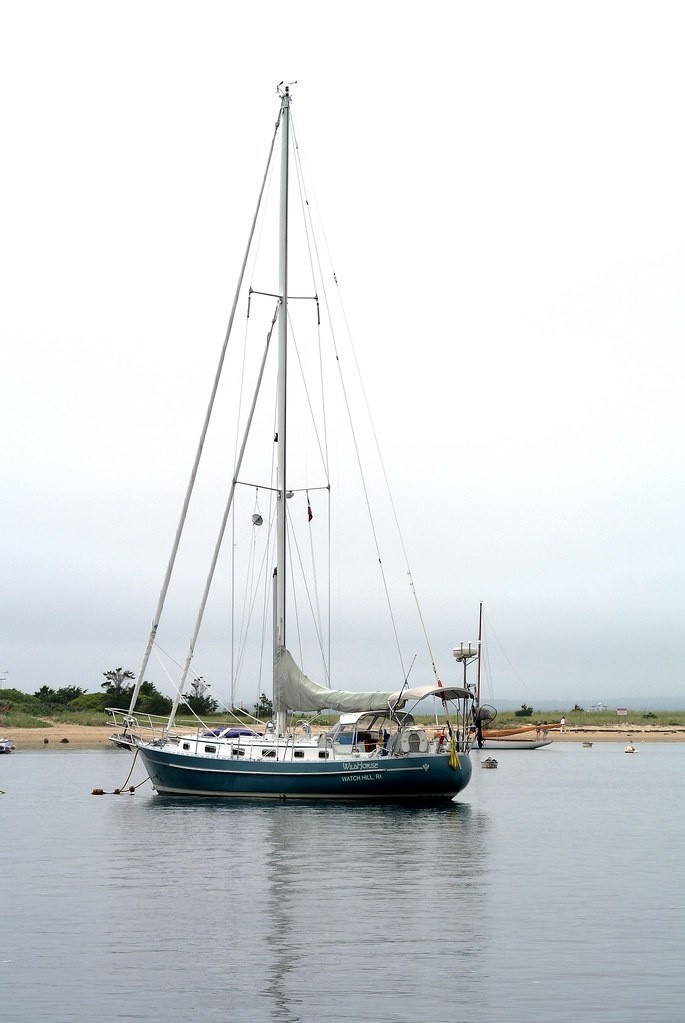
[452,601,562,749]
[104,71,477,799]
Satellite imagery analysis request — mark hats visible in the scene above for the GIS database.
[537,721,541,724]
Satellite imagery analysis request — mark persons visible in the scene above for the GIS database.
[536,721,542,738]
[354,732,363,745]
[381,729,390,756]
[561,717,566,734]
[542,721,548,739]
